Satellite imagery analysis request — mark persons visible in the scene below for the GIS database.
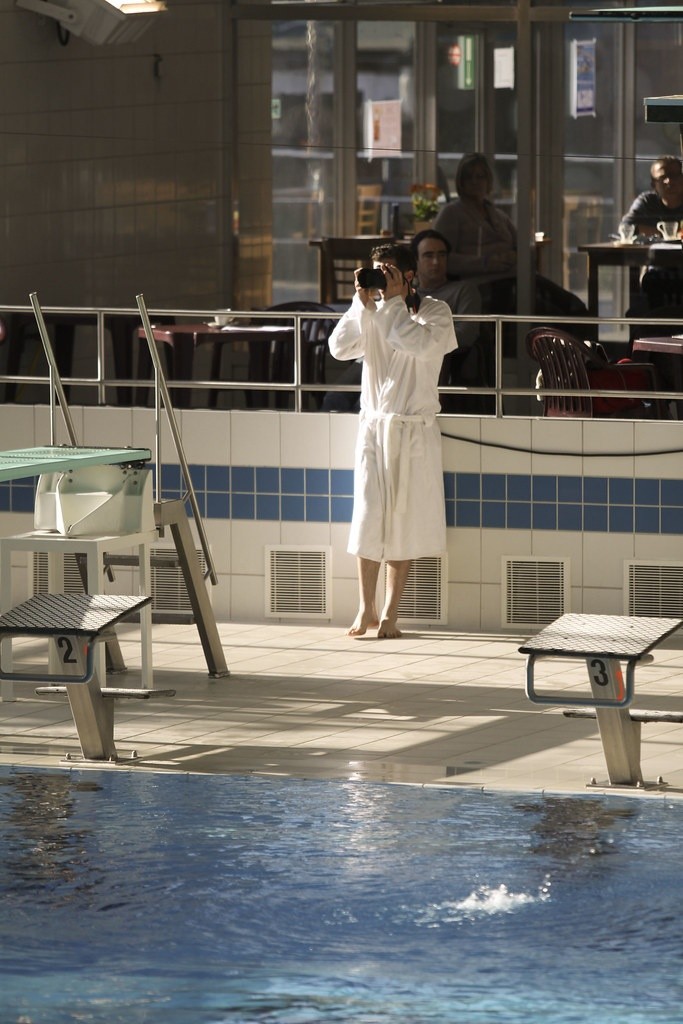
[618,156,683,356]
[323,243,462,639]
[405,225,481,399]
[432,151,589,350]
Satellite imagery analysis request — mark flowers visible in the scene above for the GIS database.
[412,196,442,223]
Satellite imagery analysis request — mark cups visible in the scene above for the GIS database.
[618,223,635,241]
[656,220,678,240]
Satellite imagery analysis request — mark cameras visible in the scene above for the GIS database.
[357,268,387,290]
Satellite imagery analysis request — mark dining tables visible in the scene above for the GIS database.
[130,321,299,407]
[5,309,157,407]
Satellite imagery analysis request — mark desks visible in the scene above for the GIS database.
[574,236,681,349]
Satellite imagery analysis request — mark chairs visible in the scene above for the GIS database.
[245,301,340,413]
[320,235,394,307]
[521,325,664,422]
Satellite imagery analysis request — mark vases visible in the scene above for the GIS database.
[411,218,435,232]
